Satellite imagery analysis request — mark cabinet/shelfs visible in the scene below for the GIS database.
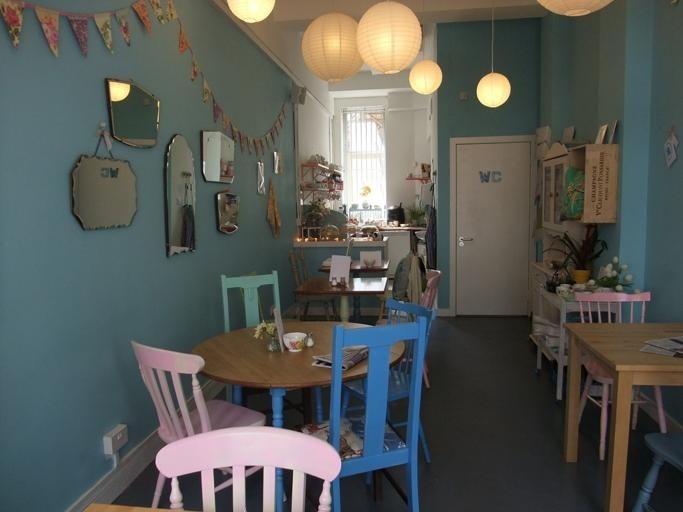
[565,144,620,224]
[530,153,568,332]
[535,287,620,402]
[301,163,344,205]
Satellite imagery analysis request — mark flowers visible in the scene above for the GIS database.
[589,258,640,294]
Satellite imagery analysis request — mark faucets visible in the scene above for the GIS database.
[365,232,372,242]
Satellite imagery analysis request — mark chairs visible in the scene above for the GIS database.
[632,433,683,512]
[289,251,338,321]
[341,299,437,463]
[378,257,426,320]
[375,269,441,388]
[131,340,287,509]
[301,316,427,512]
[574,291,667,461]
[155,426,342,512]
[221,270,304,404]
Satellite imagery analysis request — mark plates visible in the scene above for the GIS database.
[399,223,409,227]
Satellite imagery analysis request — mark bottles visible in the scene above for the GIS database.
[351,201,379,208]
[331,276,346,286]
[305,331,314,347]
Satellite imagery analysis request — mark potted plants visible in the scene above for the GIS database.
[542,223,608,283]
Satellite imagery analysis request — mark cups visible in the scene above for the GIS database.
[282,332,306,353]
[381,221,398,227]
[556,280,617,297]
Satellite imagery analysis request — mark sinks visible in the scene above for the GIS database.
[350,229,384,242]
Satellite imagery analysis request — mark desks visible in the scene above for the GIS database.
[562,322,683,512]
[193,322,405,512]
[294,277,388,322]
[319,257,390,326]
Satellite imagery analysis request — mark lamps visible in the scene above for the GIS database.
[409,60,443,95]
[537,0,615,17]
[227,0,275,24]
[301,0,364,82]
[356,0,422,74]
[476,0,511,108]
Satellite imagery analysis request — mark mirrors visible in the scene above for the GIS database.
[71,78,241,256]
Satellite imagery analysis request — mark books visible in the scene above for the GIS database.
[531,314,587,367]
[535,126,552,159]
